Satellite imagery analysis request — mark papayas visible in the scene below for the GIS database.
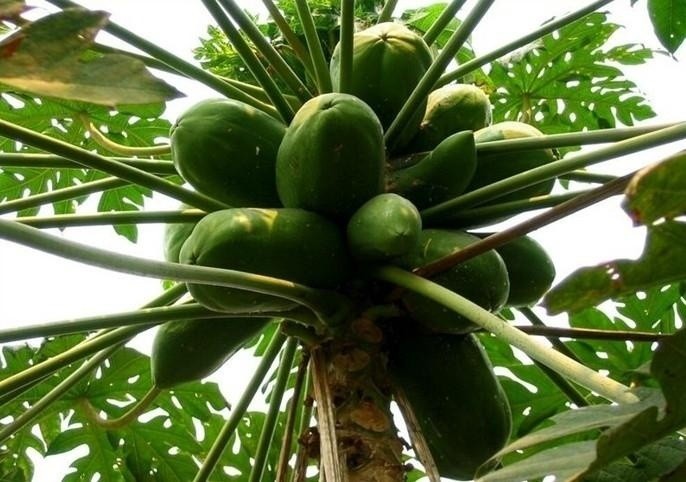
[147,20,557,481]
